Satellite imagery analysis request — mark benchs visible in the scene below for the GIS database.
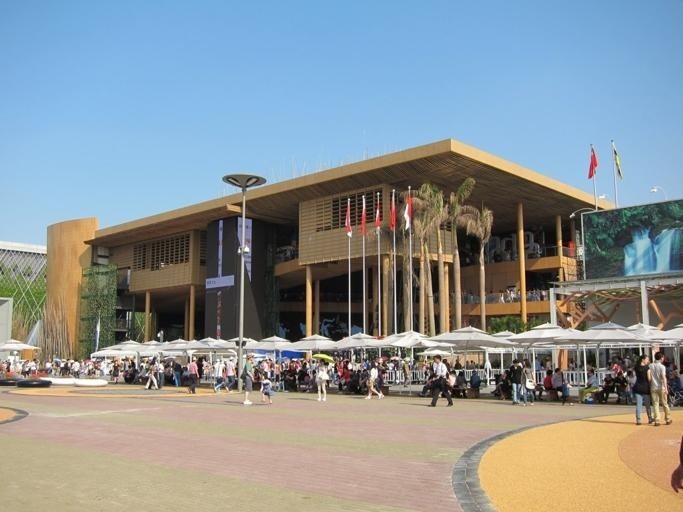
[445,387,480,400]
[361,384,393,396]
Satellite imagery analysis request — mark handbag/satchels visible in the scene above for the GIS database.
[319,373,330,381]
[525,379,536,389]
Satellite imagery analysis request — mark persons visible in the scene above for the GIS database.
[243,355,255,405]
[669,435,683,495]
[508,354,683,407]
[187,356,238,394]
[335,354,481,399]
[646,352,673,427]
[112,357,165,390]
[449,284,548,304]
[632,354,655,424]
[259,359,332,403]
[429,355,453,406]
[2,358,101,378]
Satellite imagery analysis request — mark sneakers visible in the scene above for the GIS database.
[242,400,252,404]
[429,402,453,407]
[637,420,672,426]
[365,395,384,399]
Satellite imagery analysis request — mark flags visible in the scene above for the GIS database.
[359,198,369,235]
[388,195,396,231]
[342,202,353,239]
[587,146,599,180]
[373,201,383,229]
[403,194,414,231]
[611,144,624,182]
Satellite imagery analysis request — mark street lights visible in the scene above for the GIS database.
[650,186,667,201]
[220,173,267,392]
[568,208,596,219]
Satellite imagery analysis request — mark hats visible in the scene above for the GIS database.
[245,353,254,359]
[191,356,235,362]
